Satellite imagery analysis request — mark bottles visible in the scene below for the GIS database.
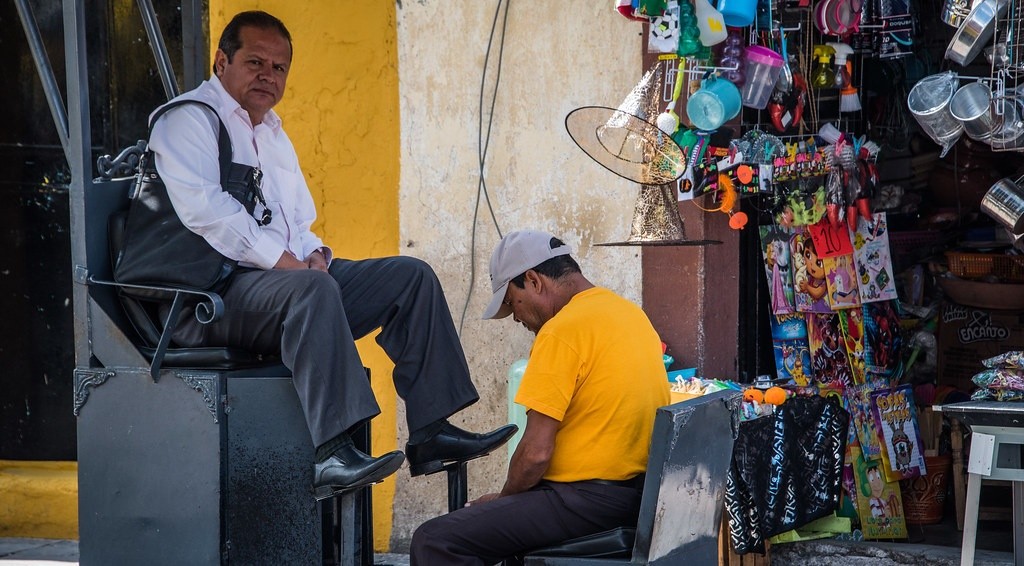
[695,0,728,47]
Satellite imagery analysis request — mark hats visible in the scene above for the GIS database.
[564,57,724,247]
[481,229,573,320]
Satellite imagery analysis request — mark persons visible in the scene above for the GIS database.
[128,10,518,501]
[409,230,671,566]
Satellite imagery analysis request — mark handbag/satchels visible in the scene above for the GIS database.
[113,100,272,291]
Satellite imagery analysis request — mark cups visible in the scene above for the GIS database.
[950,79,1002,139]
[908,73,963,144]
[640,0,668,17]
[981,176,1024,235]
[983,88,1024,148]
[717,0,758,27]
[738,46,785,109]
[686,72,742,131]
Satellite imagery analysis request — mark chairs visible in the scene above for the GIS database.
[524,389,743,566]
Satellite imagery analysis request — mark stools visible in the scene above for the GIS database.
[62,0,369,566]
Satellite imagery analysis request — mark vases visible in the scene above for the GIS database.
[899,455,948,521]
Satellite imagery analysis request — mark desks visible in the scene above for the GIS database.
[932,401,1024,566]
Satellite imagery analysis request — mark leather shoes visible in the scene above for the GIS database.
[405,422,519,477]
[311,444,404,502]
